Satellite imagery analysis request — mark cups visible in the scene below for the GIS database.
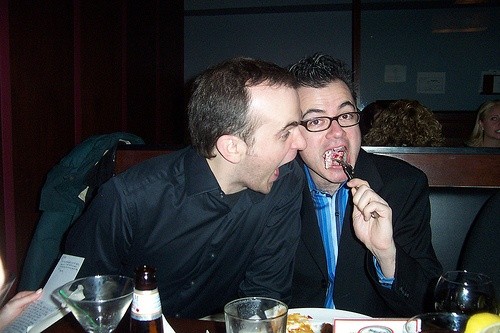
[59,274,136,333]
[223,297,289,333]
[404,270,500,333]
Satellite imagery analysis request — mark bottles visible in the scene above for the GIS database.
[128,264,164,333]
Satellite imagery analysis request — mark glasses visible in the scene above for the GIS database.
[299,111,360,133]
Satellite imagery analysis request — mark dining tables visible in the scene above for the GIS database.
[40,312,227,333]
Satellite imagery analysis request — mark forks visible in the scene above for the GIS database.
[331,158,381,219]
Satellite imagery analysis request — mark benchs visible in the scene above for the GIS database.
[92,149,500,313]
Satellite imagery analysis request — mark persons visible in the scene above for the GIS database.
[470,100,500,146]
[287,53,441,317]
[59,57,305,318]
[362,99,446,146]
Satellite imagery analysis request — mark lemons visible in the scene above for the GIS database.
[464,312,500,333]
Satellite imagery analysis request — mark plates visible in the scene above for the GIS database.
[246,308,374,333]
